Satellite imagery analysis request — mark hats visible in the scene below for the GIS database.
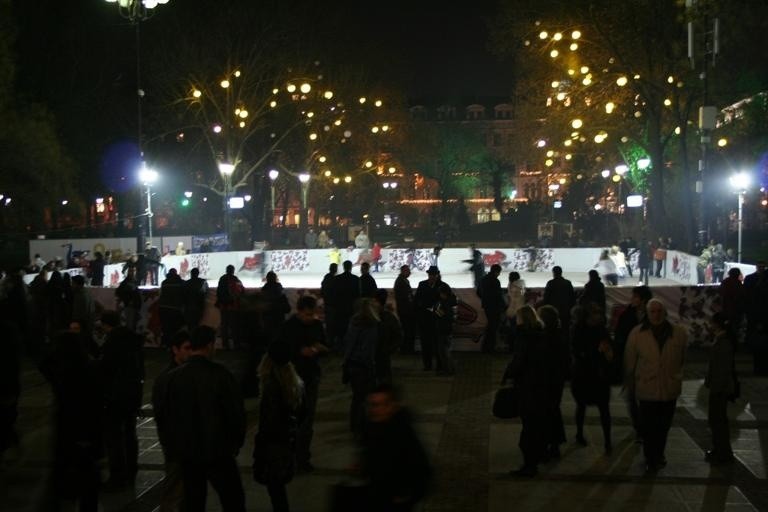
[426,265,441,274]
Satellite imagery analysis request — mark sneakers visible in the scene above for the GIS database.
[511,432,734,480]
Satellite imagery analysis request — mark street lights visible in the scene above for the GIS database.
[381,181,398,211]
[100,0,168,163]
[138,165,159,249]
[295,171,310,209]
[724,168,752,264]
[218,164,233,241]
[268,169,279,215]
